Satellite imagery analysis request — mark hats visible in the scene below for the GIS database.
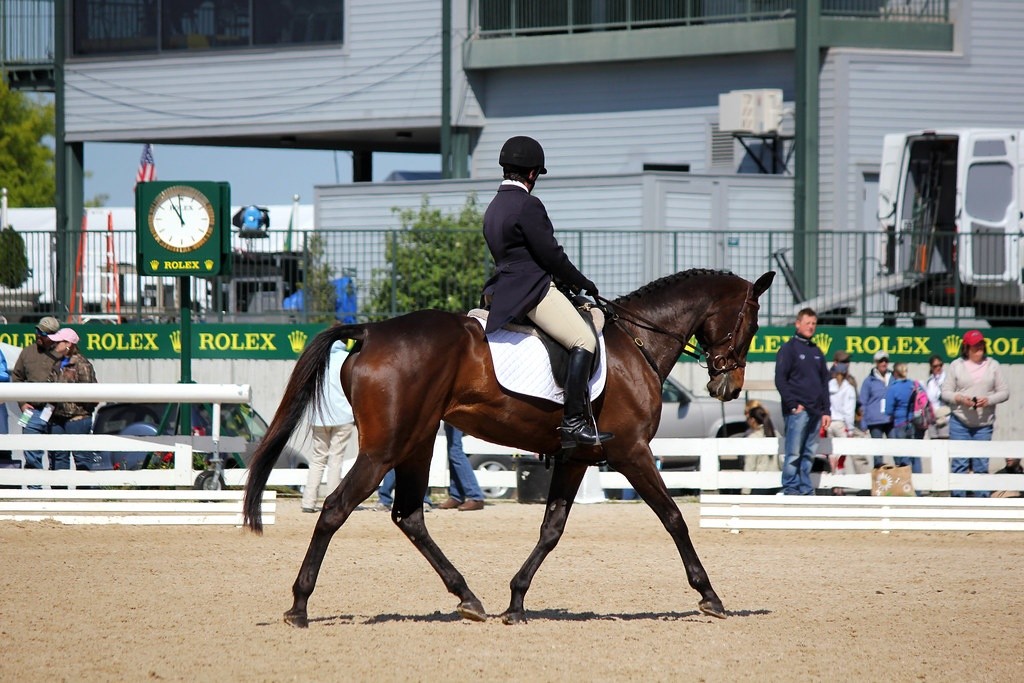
[834,351,852,360]
[834,363,848,373]
[962,330,985,345]
[874,350,889,361]
[35,316,60,332]
[48,328,79,344]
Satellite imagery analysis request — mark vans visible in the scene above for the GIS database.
[878,126,1024,321]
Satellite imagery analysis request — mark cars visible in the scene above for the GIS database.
[460,376,783,500]
[88,400,309,494]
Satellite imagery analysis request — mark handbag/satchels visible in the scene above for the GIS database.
[871,464,914,495]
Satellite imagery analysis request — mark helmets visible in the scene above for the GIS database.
[499,135,548,175]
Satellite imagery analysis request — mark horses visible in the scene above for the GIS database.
[240,268,778,625]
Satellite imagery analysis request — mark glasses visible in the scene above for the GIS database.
[932,363,943,368]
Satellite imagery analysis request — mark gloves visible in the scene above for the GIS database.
[581,279,597,298]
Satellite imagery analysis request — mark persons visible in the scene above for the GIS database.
[829,351,861,420]
[301,323,354,513]
[11,317,60,490]
[924,356,950,439]
[941,330,1009,497]
[45,328,98,490]
[885,362,926,497]
[858,350,910,467]
[775,307,831,495]
[438,421,484,511]
[622,456,665,500]
[144,0,204,50]
[480,135,614,449]
[826,362,872,495]
[374,468,433,511]
[738,400,785,495]
[0,349,11,462]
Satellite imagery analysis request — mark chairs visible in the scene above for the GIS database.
[734,106,798,174]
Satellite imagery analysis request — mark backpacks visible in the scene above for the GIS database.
[906,379,936,428]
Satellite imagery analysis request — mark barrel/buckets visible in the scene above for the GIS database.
[514,455,553,504]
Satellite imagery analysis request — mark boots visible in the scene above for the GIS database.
[557,348,615,448]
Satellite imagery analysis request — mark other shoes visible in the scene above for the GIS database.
[373,503,392,511]
[423,503,433,511]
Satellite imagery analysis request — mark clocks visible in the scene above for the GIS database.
[136,181,222,277]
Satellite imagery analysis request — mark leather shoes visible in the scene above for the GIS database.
[459,499,482,510]
[440,497,461,509]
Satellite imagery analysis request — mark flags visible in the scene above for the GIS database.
[133,143,158,192]
